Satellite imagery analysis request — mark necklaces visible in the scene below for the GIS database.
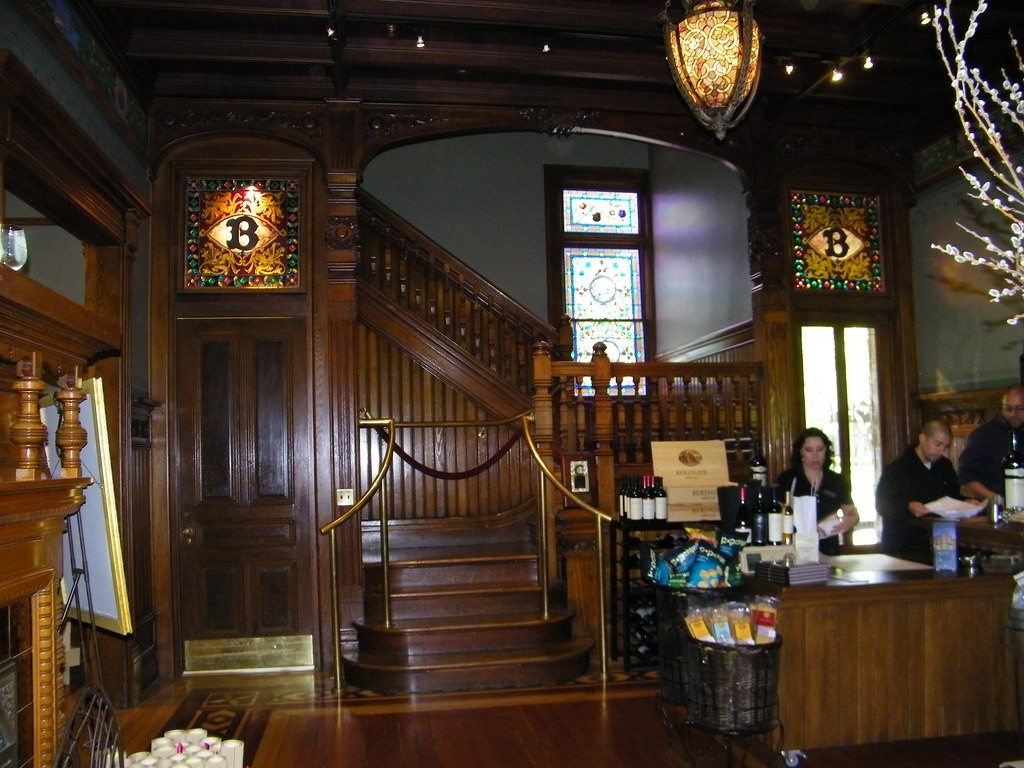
[803,467,821,482]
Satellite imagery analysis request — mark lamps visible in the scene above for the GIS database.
[660,0,770,142]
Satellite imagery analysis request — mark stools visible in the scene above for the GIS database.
[681,716,785,768]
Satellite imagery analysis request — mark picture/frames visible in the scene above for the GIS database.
[38,377,134,635]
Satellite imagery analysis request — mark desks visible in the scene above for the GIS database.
[0,469,92,768]
[903,510,1024,554]
[739,551,1021,752]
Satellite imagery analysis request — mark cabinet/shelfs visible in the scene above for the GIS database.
[609,517,683,673]
[85,399,165,709]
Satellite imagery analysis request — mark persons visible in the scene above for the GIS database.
[573,465,586,488]
[875,385,1024,553]
[773,427,860,555]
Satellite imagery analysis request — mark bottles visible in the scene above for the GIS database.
[767,485,782,545]
[618,473,668,523]
[781,491,793,545]
[752,486,768,546]
[751,440,768,487]
[1000,429,1024,512]
[735,487,752,546]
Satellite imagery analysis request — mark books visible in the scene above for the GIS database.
[756,560,829,584]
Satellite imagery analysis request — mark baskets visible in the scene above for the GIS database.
[654,582,744,706]
[688,633,783,733]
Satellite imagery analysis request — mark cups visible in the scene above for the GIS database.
[987,503,1002,525]
[93,728,244,768]
[0,223,28,271]
